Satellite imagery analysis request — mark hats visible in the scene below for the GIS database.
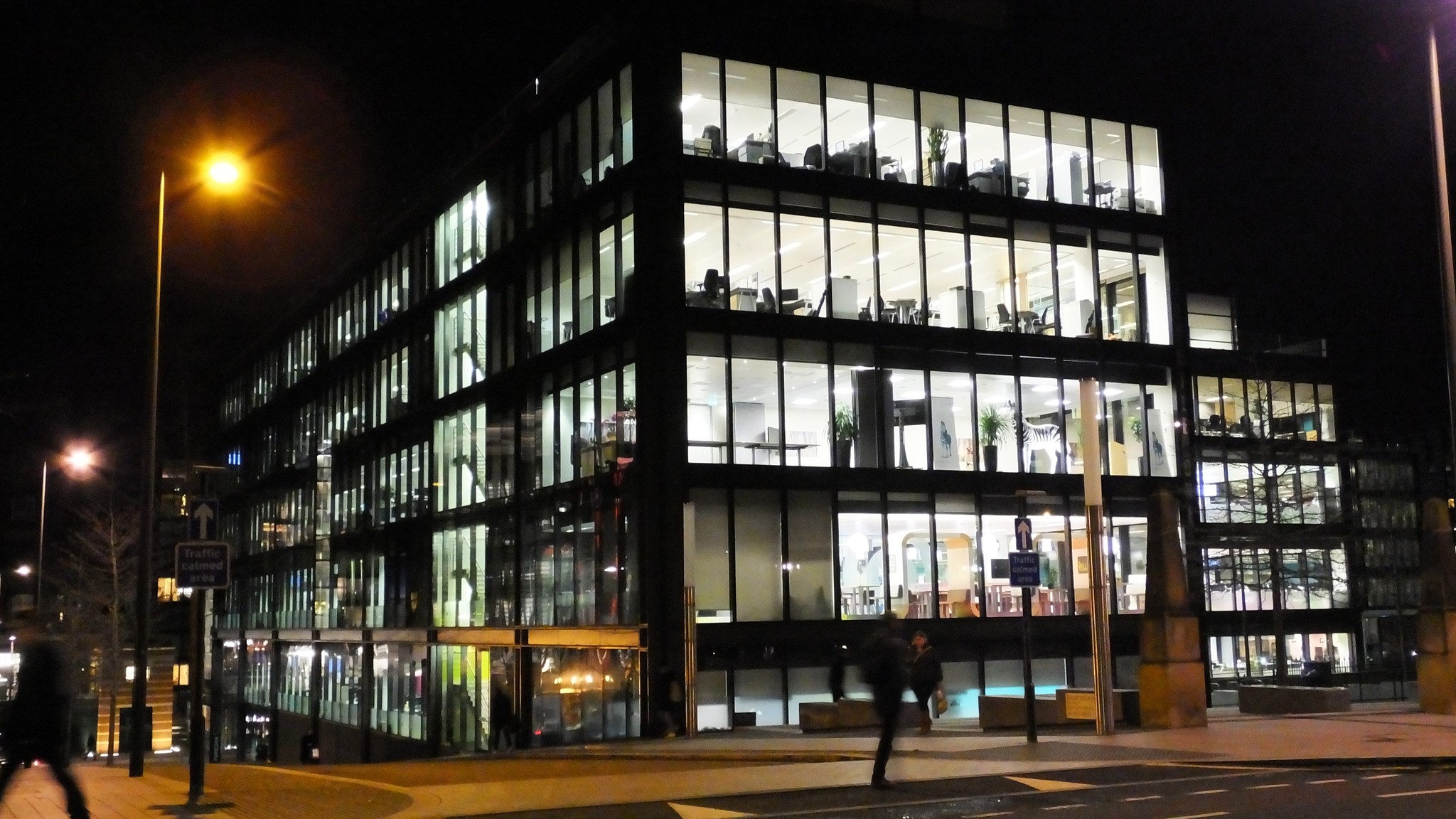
[912,636,926,652]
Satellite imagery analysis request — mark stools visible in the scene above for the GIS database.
[842,597,853,615]
[869,597,883,615]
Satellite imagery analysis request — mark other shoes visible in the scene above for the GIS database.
[920,720,933,733]
[871,778,894,790]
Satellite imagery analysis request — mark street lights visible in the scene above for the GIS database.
[128,147,254,778]
[35,441,100,629]
[1015,489,1045,744]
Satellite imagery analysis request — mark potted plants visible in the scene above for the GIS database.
[1128,413,1144,476]
[976,402,1008,472]
[1042,568,1059,589]
[928,127,949,187]
[824,403,857,468]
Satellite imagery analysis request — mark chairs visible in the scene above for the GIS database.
[762,288,776,310]
[1240,416,1250,425]
[1206,414,1221,431]
[997,304,1048,334]
[874,293,931,324]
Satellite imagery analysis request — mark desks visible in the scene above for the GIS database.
[916,591,948,618]
[830,153,894,180]
[985,584,1010,612]
[728,140,772,163]
[889,300,918,324]
[580,440,618,478]
[730,288,758,312]
[969,172,1031,197]
[850,586,880,615]
[688,440,820,466]
[1084,185,1116,208]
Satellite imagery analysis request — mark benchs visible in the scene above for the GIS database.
[940,589,970,618]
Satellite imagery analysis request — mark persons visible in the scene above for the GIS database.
[0,609,90,819]
[489,688,514,753]
[829,610,944,790]
